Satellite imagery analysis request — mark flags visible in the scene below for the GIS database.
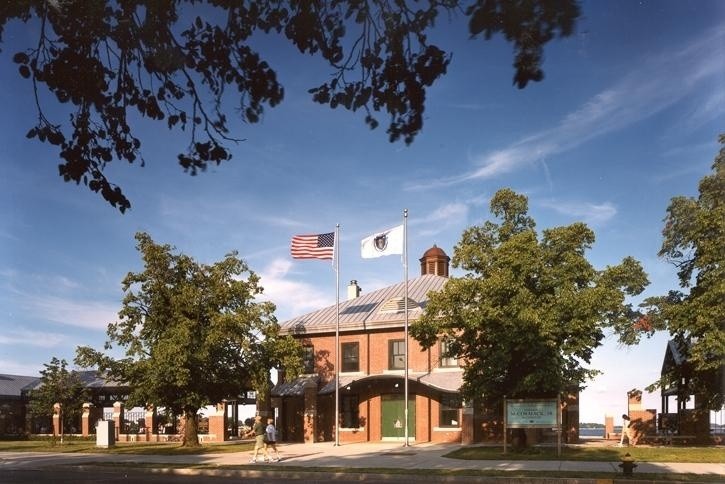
[290,231,336,261]
[360,224,405,259]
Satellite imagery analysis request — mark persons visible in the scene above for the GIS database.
[244,415,274,463]
[35,422,166,434]
[258,418,281,462]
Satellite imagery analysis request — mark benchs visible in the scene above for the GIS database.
[642,435,697,447]
